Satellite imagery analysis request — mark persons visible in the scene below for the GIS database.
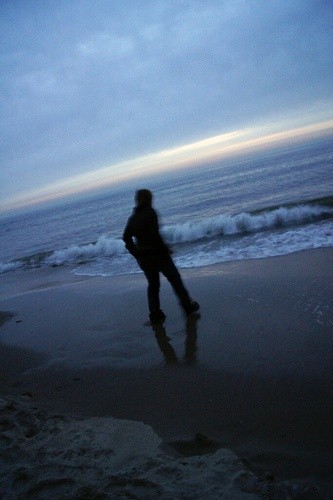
[122,189,201,324]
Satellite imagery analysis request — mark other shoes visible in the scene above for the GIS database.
[147,311,165,322]
[182,302,199,314]
